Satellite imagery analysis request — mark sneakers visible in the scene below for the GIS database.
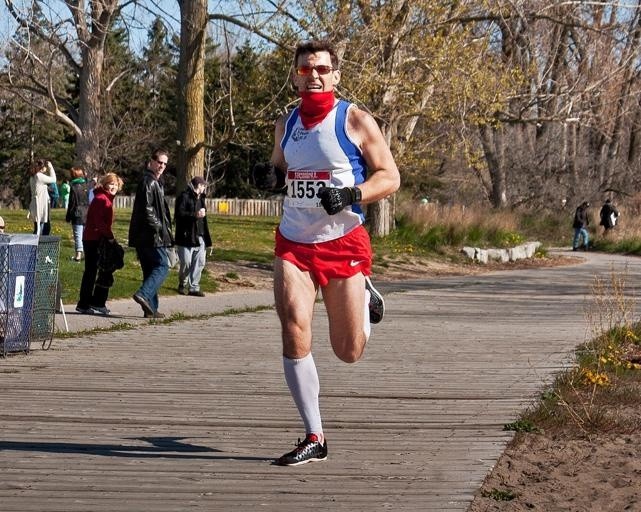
[275,434,328,466]
[134,291,154,314]
[144,311,165,318]
[76,306,94,314]
[90,305,110,314]
[188,291,205,297]
[365,275,385,326]
[71,256,80,263]
[178,288,187,295]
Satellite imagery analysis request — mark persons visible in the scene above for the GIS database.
[70,165,83,179]
[599,199,621,233]
[570,201,590,252]
[254,41,400,467]
[127,148,175,318]
[49,182,60,207]
[28,157,56,237]
[75,172,123,316]
[173,176,213,296]
[61,178,70,207]
[65,176,90,263]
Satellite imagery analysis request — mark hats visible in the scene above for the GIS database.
[192,176,209,185]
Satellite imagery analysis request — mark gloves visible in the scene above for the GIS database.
[318,186,363,216]
[255,161,279,191]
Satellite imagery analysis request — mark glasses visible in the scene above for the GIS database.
[154,159,168,165]
[294,65,338,75]
